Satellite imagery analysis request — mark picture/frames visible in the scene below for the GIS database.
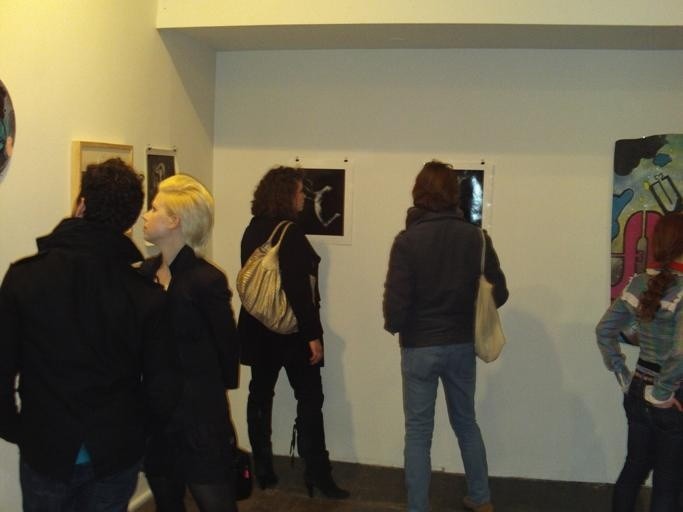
[143,143,182,249]
[72,136,137,242]
[426,158,490,234]
[280,154,356,249]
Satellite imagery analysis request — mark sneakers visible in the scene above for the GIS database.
[462,495,495,512]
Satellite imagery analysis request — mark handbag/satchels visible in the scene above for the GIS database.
[475,275,506,362]
[238,242,315,334]
[229,445,252,500]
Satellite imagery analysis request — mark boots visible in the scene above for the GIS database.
[247,396,279,487]
[295,410,349,498]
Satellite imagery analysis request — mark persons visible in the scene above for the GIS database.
[381,162,511,511]
[140,175,244,511]
[234,166,350,500]
[0,155,166,512]
[594,210,683,512]
